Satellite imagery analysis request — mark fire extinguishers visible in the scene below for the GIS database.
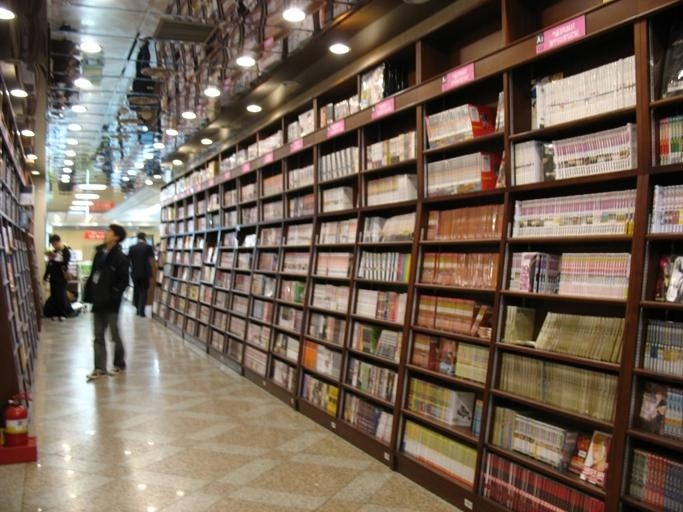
[5,392,32,446]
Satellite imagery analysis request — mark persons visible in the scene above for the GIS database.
[82,225,131,381]
[44,291,78,321]
[128,232,157,318]
[43,234,77,320]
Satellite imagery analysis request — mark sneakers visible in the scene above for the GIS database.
[50,315,69,321]
[86,369,107,379]
[109,367,125,376]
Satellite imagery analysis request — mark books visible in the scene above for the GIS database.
[0,70,39,411]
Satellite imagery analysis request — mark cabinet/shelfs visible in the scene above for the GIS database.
[0,71,46,429]
[152,34,319,411]
[318,0,683,512]
[145,0,349,176]
